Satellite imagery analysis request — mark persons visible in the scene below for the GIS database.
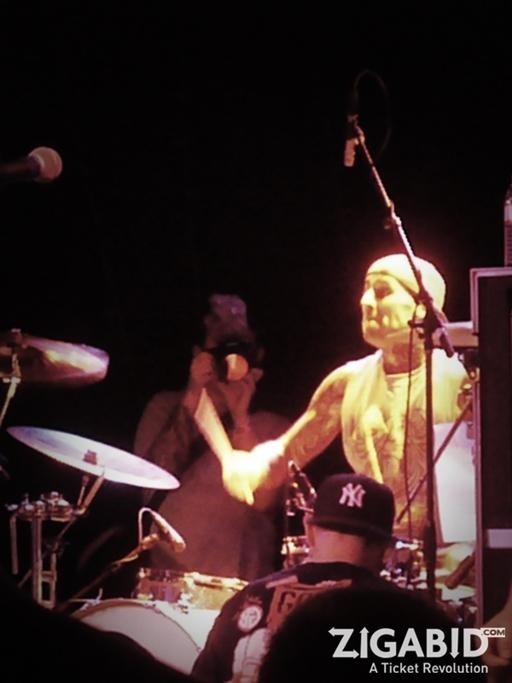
[131,294,293,584]
[188,474,403,683]
[217,254,475,591]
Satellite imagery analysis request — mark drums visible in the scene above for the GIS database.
[134,568,251,607]
[402,573,477,623]
[66,600,250,682]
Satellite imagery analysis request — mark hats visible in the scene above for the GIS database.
[366,252,448,313]
[307,473,398,545]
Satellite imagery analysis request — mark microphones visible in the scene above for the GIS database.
[0,145,63,184]
[288,460,317,504]
[147,508,187,553]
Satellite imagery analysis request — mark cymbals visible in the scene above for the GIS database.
[0,334,108,386]
[6,423,182,492]
[386,318,479,348]
